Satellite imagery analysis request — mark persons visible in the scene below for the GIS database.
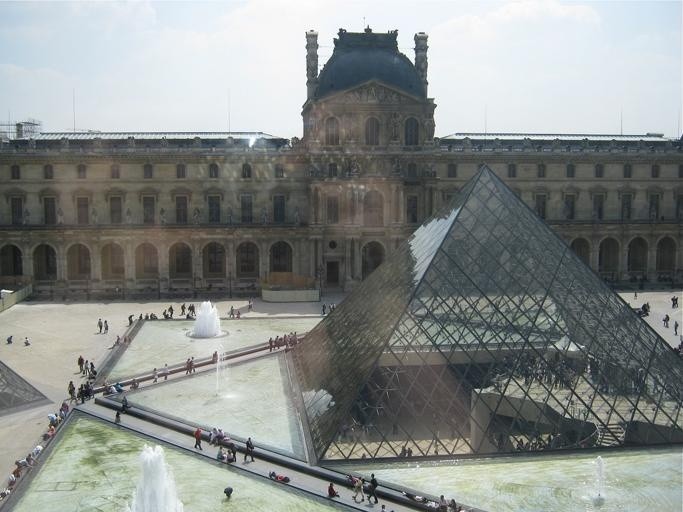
[0,355,99,512]
[338,412,457,458]
[6,336,13,344]
[328,474,457,512]
[25,336,30,346]
[269,331,298,352]
[194,427,290,484]
[617,293,683,353]
[502,352,683,447]
[93,300,253,349]
[323,303,337,314]
[101,350,218,424]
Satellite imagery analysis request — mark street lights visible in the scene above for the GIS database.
[47,273,56,302]
[228,270,233,299]
[316,264,324,297]
[85,272,90,301]
